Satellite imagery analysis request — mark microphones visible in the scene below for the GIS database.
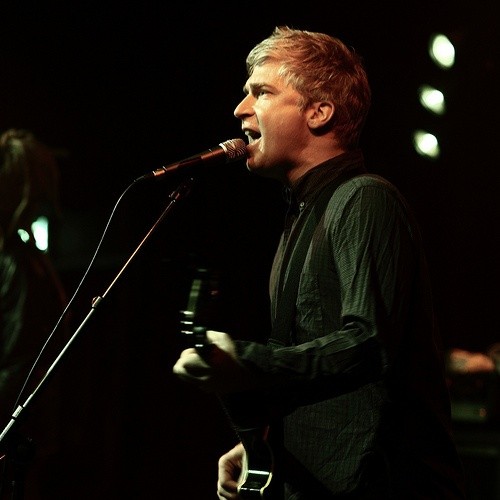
[133,139,246,181]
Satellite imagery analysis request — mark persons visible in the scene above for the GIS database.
[0,127,70,500]
[171,26,459,500]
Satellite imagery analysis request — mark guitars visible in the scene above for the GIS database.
[180,267,286,500]
